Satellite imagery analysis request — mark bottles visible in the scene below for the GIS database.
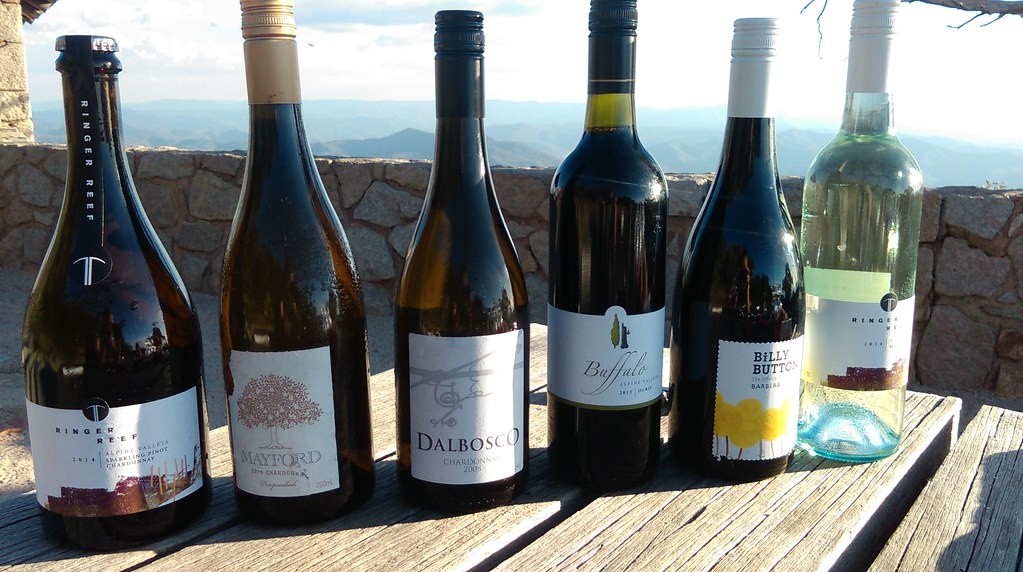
[672,18,804,483]
[393,9,529,512]
[547,0,670,494]
[21,35,213,551]
[217,0,375,527]
[798,0,924,462]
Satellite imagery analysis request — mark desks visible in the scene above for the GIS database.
[0,319,1023,572]
